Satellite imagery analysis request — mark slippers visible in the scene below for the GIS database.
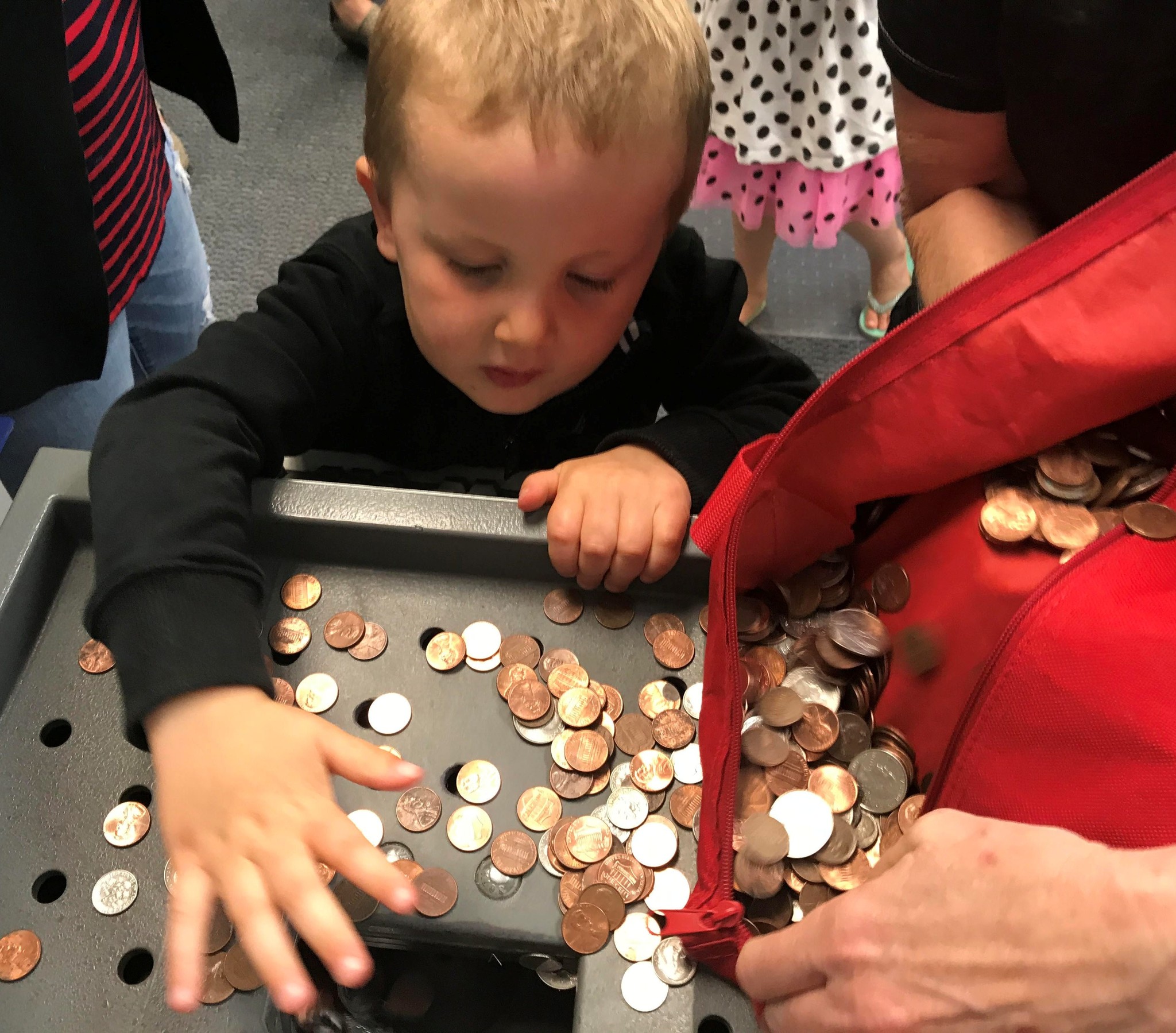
[739,300,767,326]
[858,241,915,340]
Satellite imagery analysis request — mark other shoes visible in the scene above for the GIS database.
[329,0,381,62]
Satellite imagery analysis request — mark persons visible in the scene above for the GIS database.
[879,0,1176,335]
[328,0,382,60]
[0,0,239,500]
[87,0,823,1014]
[736,808,1176,1033]
[690,0,913,333]
